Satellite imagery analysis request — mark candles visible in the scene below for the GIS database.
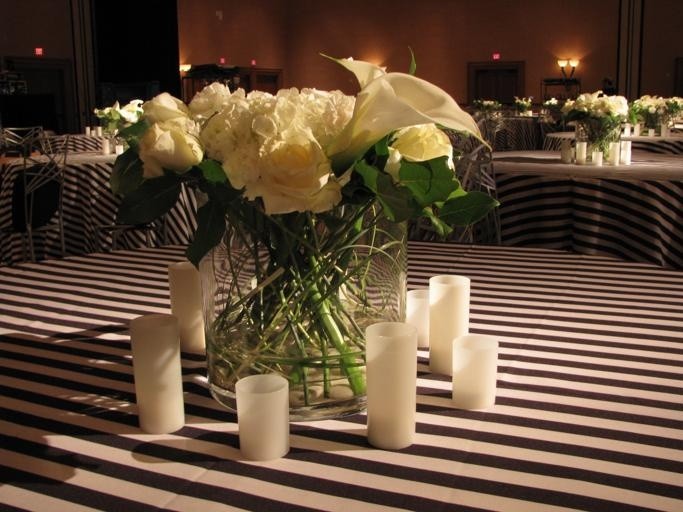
[35,48,43,56]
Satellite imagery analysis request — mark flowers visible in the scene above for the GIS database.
[96,54,501,242]
[514,95,536,111]
[544,91,683,146]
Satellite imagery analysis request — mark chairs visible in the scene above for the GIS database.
[3,127,53,154]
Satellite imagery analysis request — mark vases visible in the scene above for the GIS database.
[574,121,619,164]
[191,183,413,425]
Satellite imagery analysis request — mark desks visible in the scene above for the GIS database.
[0,134,683,512]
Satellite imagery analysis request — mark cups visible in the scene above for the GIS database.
[84,124,126,158]
[405,287,431,350]
[234,373,291,461]
[129,314,185,435]
[168,259,208,352]
[519,108,553,118]
[558,120,673,167]
[363,320,418,452]
[428,274,472,377]
[451,333,499,411]
